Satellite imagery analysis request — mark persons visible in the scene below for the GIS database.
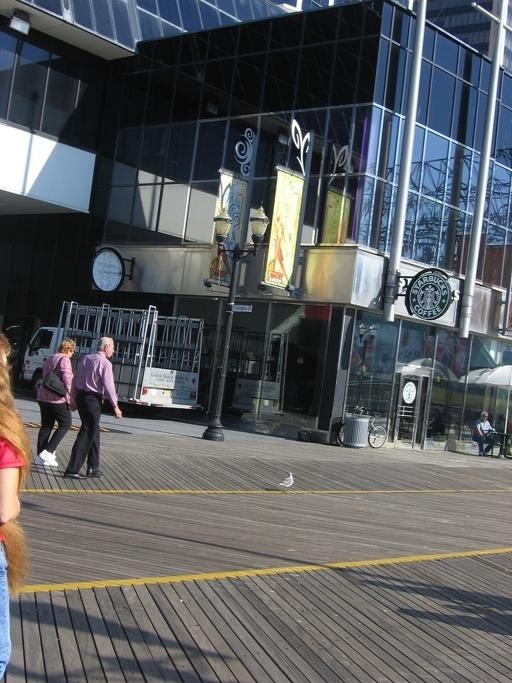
[474,410,498,456]
[32,337,77,468]
[0,329,33,681]
[425,406,446,437]
[60,335,124,480]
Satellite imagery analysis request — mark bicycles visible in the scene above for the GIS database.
[337,405,387,448]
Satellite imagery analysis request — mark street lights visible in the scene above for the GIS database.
[202,204,270,441]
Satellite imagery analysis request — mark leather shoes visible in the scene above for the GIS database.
[63,471,89,479]
[86,466,105,476]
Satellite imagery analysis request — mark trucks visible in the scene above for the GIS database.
[19,300,204,416]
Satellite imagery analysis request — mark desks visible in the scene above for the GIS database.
[489,430,511,458]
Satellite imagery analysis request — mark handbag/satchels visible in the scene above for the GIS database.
[42,355,69,398]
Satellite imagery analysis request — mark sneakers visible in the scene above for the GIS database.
[39,449,58,467]
[34,454,51,466]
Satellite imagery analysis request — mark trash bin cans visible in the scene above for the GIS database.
[343,413,370,448]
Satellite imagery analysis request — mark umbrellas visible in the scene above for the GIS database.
[458,366,492,409]
[474,362,512,434]
[394,356,459,438]
